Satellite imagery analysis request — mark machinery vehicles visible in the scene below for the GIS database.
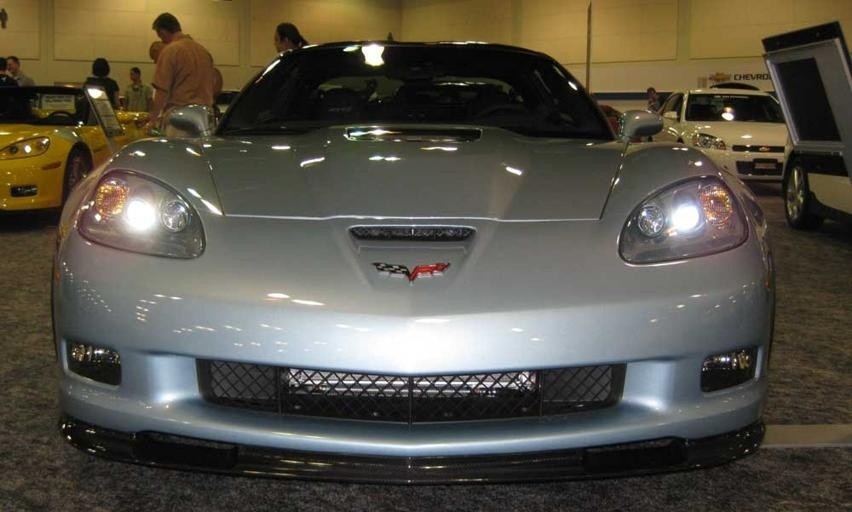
[45,31,780,487]
[0,80,157,230]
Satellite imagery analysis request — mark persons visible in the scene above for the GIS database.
[143,14,219,137]
[7,56,35,86]
[0,57,18,86]
[123,67,154,113]
[273,21,309,52]
[149,41,165,64]
[646,88,666,113]
[85,57,123,111]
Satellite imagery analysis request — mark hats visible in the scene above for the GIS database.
[781,129,852,235]
[646,82,795,188]
[209,87,242,120]
